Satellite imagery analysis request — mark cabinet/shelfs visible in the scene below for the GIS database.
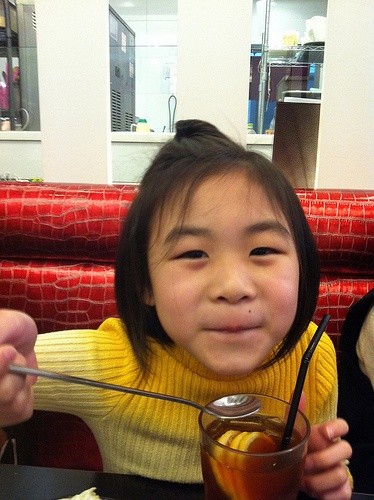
[109,6,136,133]
[16,4,40,131]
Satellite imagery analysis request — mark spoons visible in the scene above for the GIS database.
[7,362,262,420]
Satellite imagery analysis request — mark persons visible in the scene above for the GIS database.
[278,16,325,104]
[336,287,374,500]
[0,118,354,500]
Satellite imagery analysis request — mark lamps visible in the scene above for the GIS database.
[305,14,327,43]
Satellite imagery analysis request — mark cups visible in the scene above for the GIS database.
[197,392,310,500]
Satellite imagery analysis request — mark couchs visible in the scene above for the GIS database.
[0,181,374,472]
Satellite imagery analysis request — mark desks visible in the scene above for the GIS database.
[111,131,273,188]
[0,464,374,500]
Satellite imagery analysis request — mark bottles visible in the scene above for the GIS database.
[135,119,150,132]
[247,123,256,134]
